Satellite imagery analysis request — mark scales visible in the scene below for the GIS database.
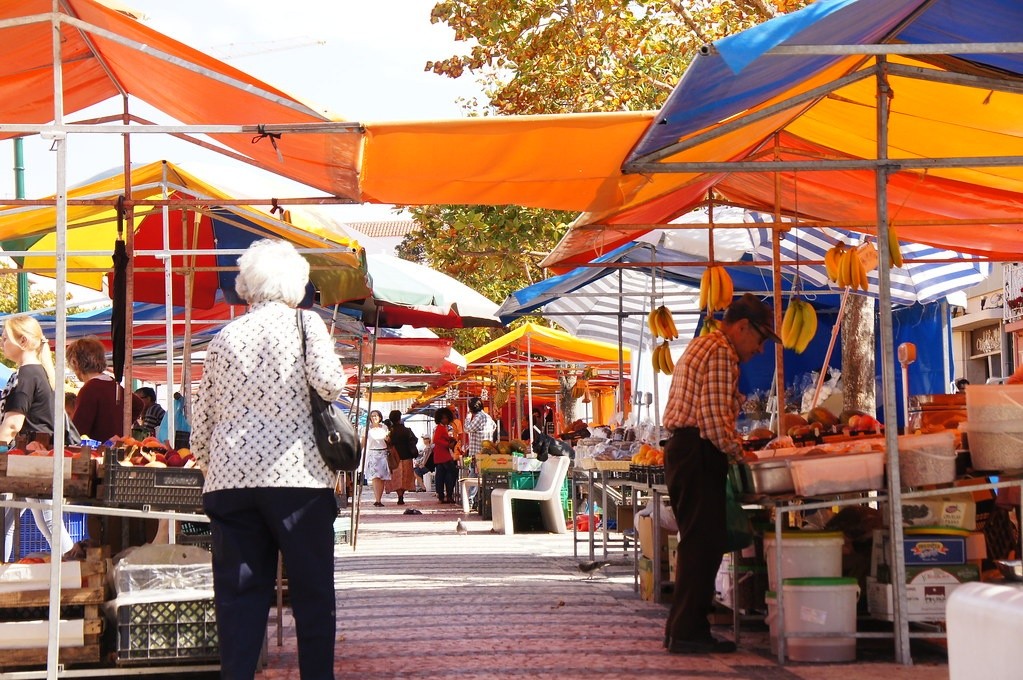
[899,343,919,435]
[644,391,662,431]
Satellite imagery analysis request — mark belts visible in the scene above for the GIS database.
[658,428,698,446]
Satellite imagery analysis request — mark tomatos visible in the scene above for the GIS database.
[6,436,194,458]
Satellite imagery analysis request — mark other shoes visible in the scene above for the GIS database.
[398,496,404,506]
[469,499,473,510]
[438,494,444,502]
[662,631,737,654]
[417,487,426,492]
[448,496,455,504]
[374,502,384,508]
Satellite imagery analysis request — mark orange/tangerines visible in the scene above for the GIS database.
[631,443,665,467]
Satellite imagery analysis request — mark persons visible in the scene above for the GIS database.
[955,378,970,395]
[66,339,145,544]
[134,387,166,431]
[0,316,74,562]
[408,434,432,492]
[362,410,390,507]
[189,238,345,680]
[389,410,418,504]
[464,397,496,511]
[433,405,462,504]
[66,393,76,419]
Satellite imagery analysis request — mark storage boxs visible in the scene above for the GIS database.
[474,394,1023,624]
[0,433,225,666]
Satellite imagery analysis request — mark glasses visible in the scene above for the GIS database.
[748,319,769,346]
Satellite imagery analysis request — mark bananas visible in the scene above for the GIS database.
[700,316,722,336]
[698,265,734,317]
[651,340,675,375]
[648,306,678,341]
[825,223,902,292]
[781,297,817,354]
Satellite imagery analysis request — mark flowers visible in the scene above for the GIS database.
[1006,288,1023,311]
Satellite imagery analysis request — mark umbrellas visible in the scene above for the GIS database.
[112,195,126,405]
[661,293,782,651]
[353,254,505,550]
[542,260,702,404]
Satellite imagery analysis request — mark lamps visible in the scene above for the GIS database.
[981,295,989,310]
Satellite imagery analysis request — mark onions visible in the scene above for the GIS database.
[117,439,198,469]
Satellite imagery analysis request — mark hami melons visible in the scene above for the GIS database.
[480,439,528,454]
[748,406,866,440]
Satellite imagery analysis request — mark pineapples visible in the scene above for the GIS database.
[495,373,514,407]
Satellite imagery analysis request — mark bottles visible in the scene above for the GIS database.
[0,441,8,453]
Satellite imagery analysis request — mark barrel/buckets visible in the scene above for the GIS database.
[946,581,1023,680]
[964,384,1023,422]
[763,531,845,592]
[899,432,955,486]
[957,422,1023,470]
[765,591,778,656]
[782,577,861,662]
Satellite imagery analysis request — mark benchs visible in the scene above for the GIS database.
[490,456,570,535]
[457,473,505,513]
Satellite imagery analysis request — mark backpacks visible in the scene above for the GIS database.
[425,448,437,472]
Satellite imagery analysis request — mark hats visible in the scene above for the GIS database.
[422,434,431,442]
[729,293,783,344]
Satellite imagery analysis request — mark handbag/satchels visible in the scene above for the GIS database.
[158,395,190,443]
[295,307,361,471]
[386,442,399,469]
[407,428,418,458]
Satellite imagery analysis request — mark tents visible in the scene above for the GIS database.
[1,0,656,680]
[0,159,464,545]
[408,324,631,440]
[492,0,1023,664]
[0,159,466,538]
